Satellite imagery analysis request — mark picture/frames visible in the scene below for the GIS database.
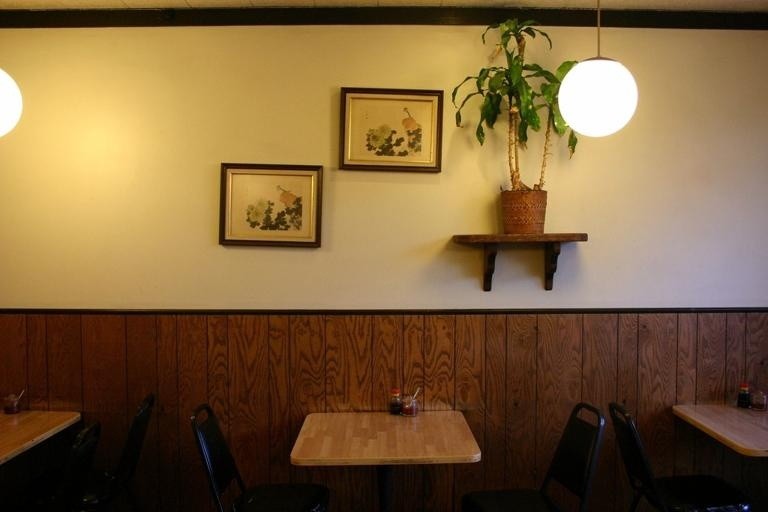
[218,162,323,251]
[338,85,445,175]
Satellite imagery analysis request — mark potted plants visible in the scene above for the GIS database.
[452,5,578,235]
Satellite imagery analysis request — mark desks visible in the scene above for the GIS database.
[0,409,82,468]
[672,400,768,462]
[288,411,483,510]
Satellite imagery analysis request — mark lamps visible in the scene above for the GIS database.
[556,1,641,137]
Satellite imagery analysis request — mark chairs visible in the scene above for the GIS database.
[188,402,330,510]
[610,401,752,510]
[16,393,155,510]
[462,403,605,512]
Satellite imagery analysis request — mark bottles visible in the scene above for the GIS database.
[736,383,767,411]
[3,394,21,414]
[389,388,419,417]
